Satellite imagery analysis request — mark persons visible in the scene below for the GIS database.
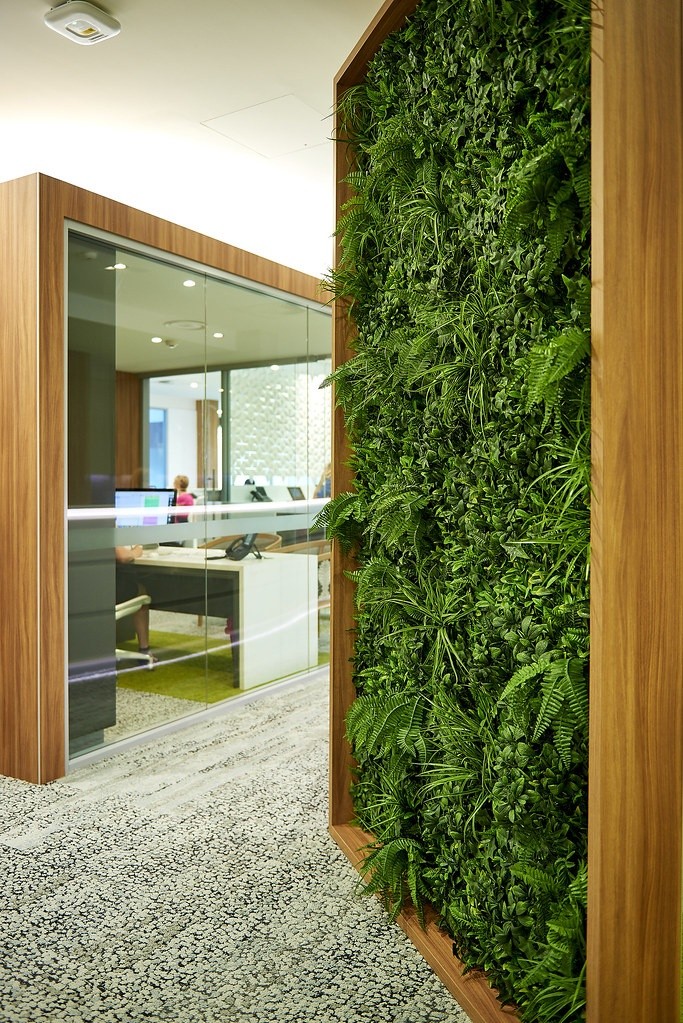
[116,545,159,666]
[313,463,331,499]
[173,475,194,523]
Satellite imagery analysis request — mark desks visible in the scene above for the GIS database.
[120,545,319,690]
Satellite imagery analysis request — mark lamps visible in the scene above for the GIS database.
[44,0,122,46]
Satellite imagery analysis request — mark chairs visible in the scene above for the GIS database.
[182,496,207,547]
[272,539,332,622]
[115,595,154,671]
[197,532,282,627]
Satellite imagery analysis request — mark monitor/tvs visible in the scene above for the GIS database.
[287,486,305,501]
[114,487,178,550]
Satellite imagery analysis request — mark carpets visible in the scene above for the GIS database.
[116,629,330,704]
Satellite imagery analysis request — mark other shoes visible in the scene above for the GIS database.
[138,649,159,664]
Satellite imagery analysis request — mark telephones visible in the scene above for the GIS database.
[225,533,257,561]
[250,487,273,502]
